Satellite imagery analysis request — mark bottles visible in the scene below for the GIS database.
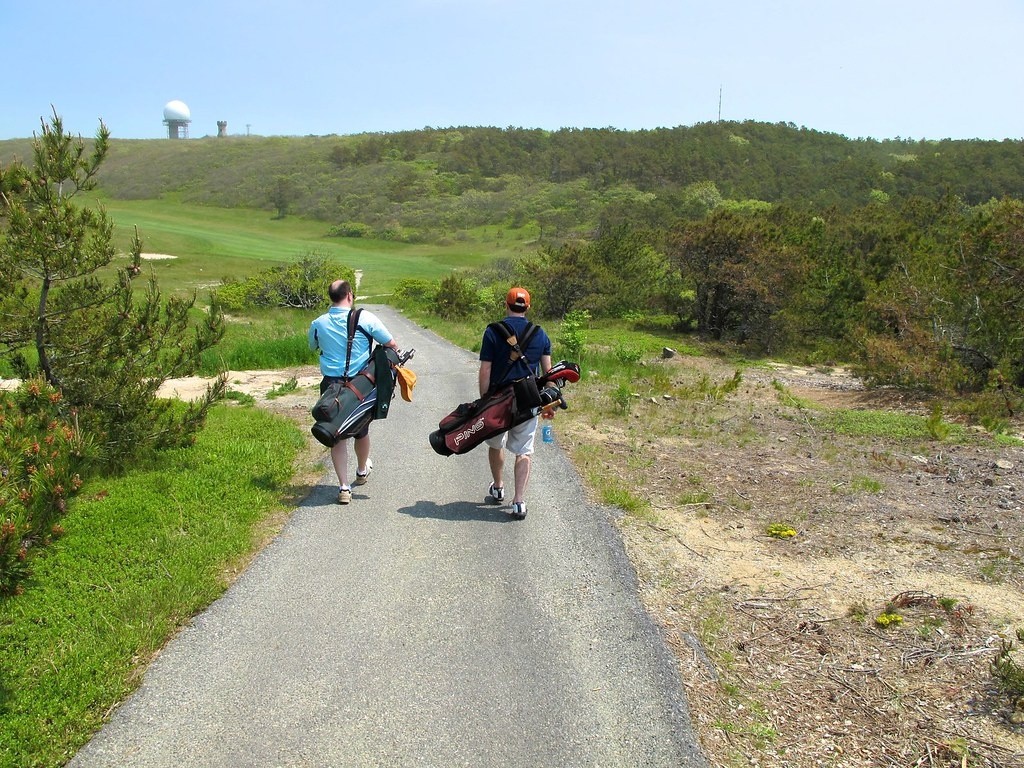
[540,413,553,443]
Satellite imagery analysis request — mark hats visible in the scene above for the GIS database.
[507,288,530,307]
[395,365,416,402]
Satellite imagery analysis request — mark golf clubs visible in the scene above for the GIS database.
[392,346,416,366]
[539,386,568,410]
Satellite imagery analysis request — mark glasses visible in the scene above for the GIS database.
[348,292,355,298]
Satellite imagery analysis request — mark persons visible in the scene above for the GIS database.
[478,287,553,520]
[308,280,397,504]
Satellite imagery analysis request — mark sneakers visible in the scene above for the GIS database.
[356,458,372,484]
[338,489,352,503]
[512,502,527,518]
[490,481,504,501]
